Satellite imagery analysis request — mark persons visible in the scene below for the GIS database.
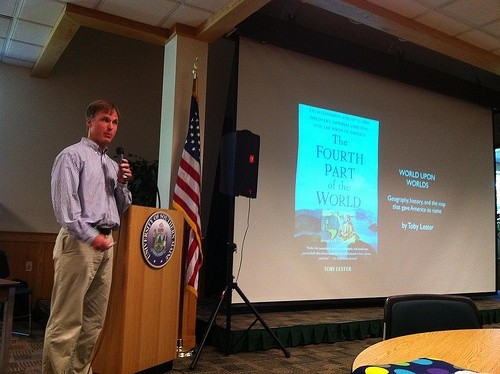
[41,99,133,374]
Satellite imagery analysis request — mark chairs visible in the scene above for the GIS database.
[0,250,33,337]
[382,295,483,343]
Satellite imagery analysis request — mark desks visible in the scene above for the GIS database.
[0,280,19,374]
[351,329,500,374]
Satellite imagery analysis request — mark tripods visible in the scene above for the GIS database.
[190,193,291,370]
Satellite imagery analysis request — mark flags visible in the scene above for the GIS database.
[171,78,204,298]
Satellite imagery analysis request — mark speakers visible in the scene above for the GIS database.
[219,130,260,198]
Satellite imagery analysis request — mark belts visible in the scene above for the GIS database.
[96,228,112,234]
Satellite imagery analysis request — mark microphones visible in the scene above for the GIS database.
[116,147,127,179]
